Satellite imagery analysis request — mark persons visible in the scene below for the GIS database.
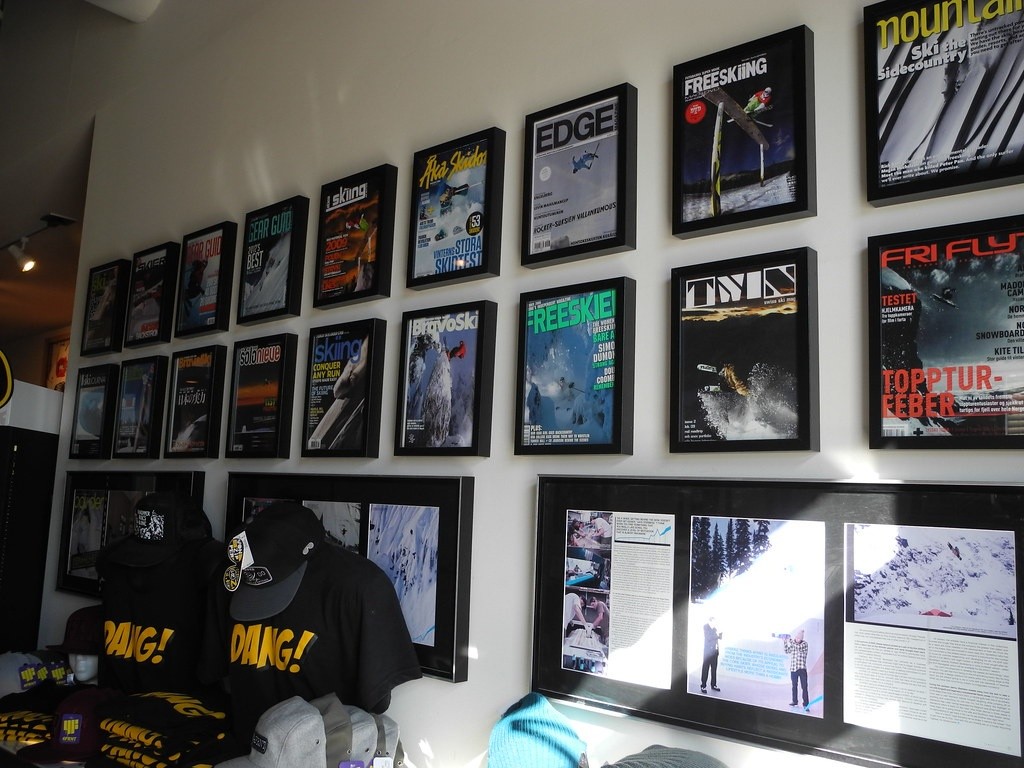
[572,153,594,173]
[440,184,469,216]
[784,629,810,711]
[744,87,772,117]
[701,617,723,694]
[44,620,99,682]
[564,515,612,644]
[449,341,466,359]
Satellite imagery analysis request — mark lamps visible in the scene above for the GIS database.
[8,236,35,274]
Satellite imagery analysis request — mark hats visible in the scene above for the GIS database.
[108,494,211,568]
[46,606,106,655]
[228,502,323,622]
[0,649,77,701]
[19,683,122,764]
[212,692,404,768]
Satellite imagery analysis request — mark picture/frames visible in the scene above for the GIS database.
[234,194,310,328]
[672,23,816,240]
[173,220,239,340]
[521,82,640,270]
[866,214,1024,452]
[79,258,131,358]
[299,317,387,458]
[311,163,398,310]
[163,344,228,460]
[44,337,69,392]
[862,0,1024,208]
[392,299,498,459]
[224,332,298,460]
[54,470,207,601]
[223,469,477,687]
[669,244,821,455]
[404,127,506,294]
[67,362,120,461]
[530,471,1024,768]
[513,276,636,457]
[110,355,170,460]
[123,240,181,350]
